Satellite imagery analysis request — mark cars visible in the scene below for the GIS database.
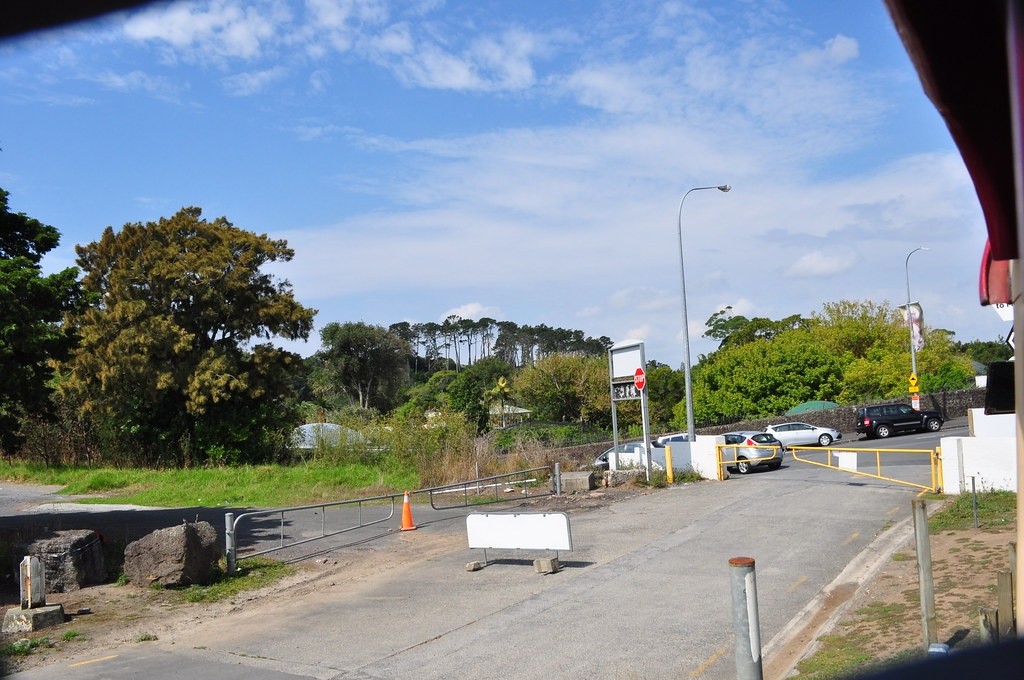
[718,432,784,473]
[594,432,703,470]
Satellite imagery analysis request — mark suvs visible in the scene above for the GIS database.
[764,422,843,450]
[854,403,943,439]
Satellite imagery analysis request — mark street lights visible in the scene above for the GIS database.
[677,184,733,442]
[905,247,933,393]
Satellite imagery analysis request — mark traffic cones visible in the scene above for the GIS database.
[399,490,417,531]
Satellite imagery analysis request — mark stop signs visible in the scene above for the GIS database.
[634,367,646,391]
